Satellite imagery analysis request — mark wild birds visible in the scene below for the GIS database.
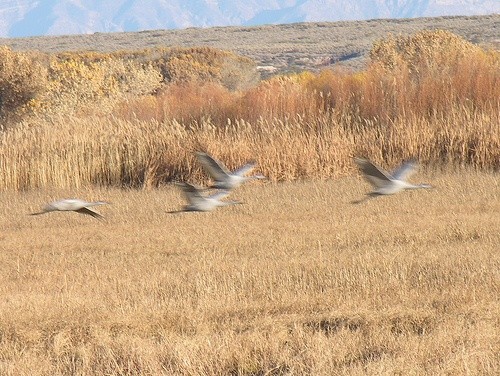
[25,200,113,223]
[163,177,245,213]
[193,149,267,191]
[350,151,436,204]
